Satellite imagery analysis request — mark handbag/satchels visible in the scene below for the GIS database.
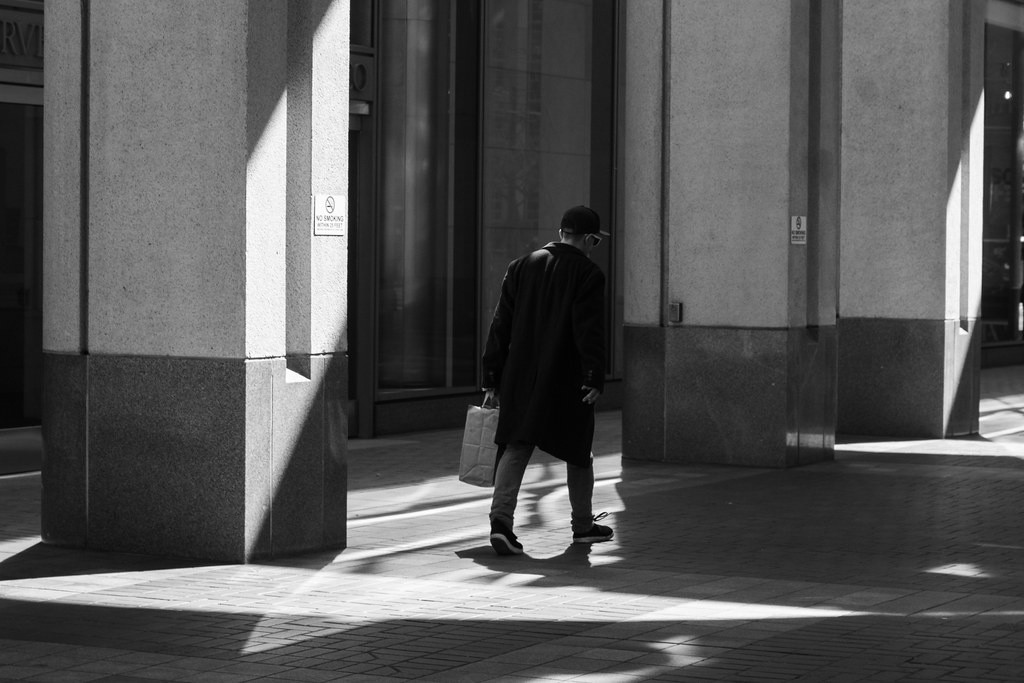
[459,390,501,489]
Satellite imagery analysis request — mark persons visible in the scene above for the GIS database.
[480,206,614,552]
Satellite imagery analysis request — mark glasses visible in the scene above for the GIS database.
[585,233,602,247]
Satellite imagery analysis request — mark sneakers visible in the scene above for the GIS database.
[490,522,524,554]
[572,512,614,544]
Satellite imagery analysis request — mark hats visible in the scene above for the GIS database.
[561,205,611,237]
